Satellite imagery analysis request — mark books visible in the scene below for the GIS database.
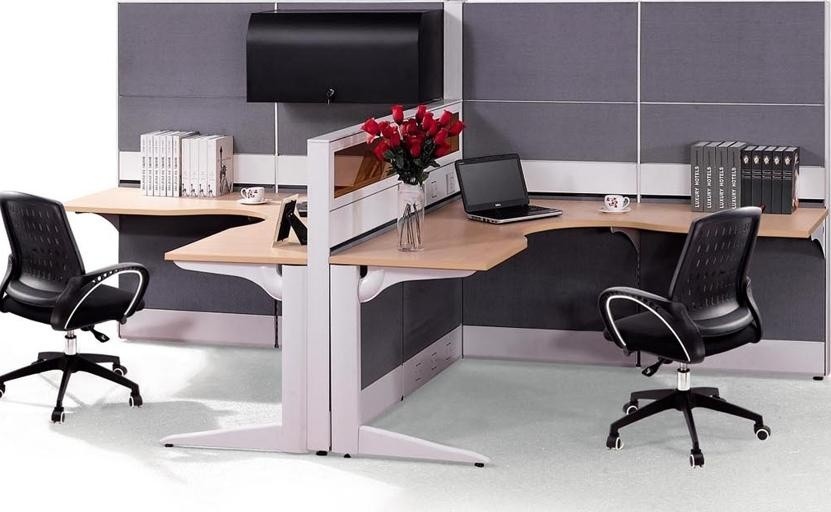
[139,129,235,199]
[690,141,802,215]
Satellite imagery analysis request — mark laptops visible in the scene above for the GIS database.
[455,153,563,225]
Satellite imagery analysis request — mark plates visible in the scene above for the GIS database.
[598,206,632,214]
[238,198,269,205]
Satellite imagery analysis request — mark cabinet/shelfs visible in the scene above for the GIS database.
[246,9,443,104]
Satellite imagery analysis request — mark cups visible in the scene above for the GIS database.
[240,186,265,202]
[604,195,631,211]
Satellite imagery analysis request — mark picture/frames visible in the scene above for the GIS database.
[272,193,308,248]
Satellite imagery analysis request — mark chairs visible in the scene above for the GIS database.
[1,191,151,423]
[597,206,771,466]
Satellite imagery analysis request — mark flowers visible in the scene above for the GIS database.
[360,104,466,187]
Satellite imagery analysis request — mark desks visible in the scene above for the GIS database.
[329,197,830,467]
[64,186,309,456]
